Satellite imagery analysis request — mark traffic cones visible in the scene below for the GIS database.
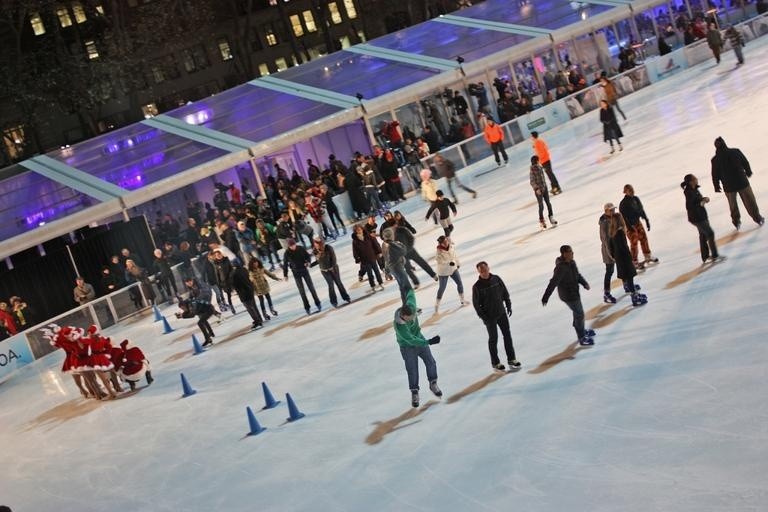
[151,306,161,319]
[262,381,280,408]
[160,317,174,335]
[189,335,203,355]
[176,373,193,397]
[247,408,264,436]
[288,393,304,424]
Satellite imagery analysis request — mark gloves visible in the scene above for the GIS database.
[428,335,440,345]
[449,261,455,267]
[507,308,512,317]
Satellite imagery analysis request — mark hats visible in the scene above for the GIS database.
[604,202,618,211]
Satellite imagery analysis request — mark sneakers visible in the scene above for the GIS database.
[549,216,559,225]
[429,379,443,399]
[201,312,224,345]
[251,307,277,328]
[413,284,420,290]
[580,337,594,345]
[433,273,439,282]
[604,294,616,304]
[539,220,546,230]
[585,329,596,336]
[624,284,640,293]
[493,363,505,372]
[508,359,521,369]
[631,292,647,306]
[411,389,419,406]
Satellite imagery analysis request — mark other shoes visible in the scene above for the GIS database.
[632,263,645,270]
[711,255,727,262]
[645,255,659,264]
[460,300,470,305]
[702,255,712,263]
[435,303,439,312]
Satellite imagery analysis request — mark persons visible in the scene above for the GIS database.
[630,0,768,65]
[391,292,443,410]
[710,137,765,231]
[472,261,520,372]
[0,209,171,401]
[599,201,642,304]
[540,245,596,346]
[680,174,729,264]
[344,81,509,315]
[609,213,647,306]
[172,153,350,348]
[619,183,658,270]
[495,47,643,229]
